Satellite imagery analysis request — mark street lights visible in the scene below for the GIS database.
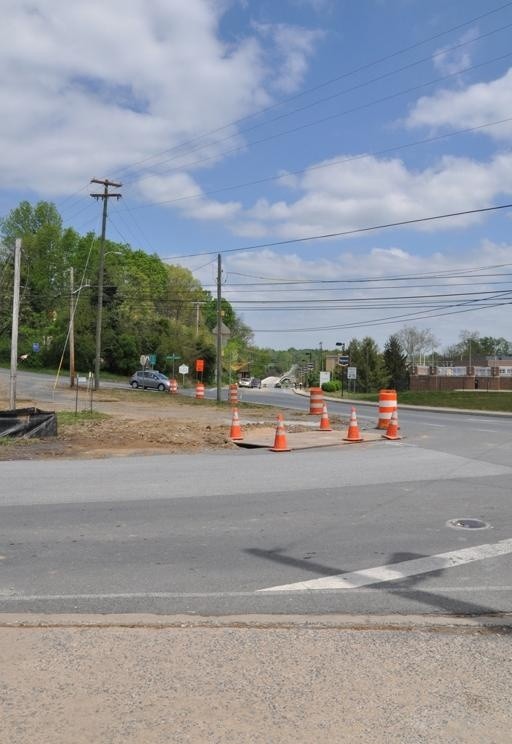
[302,359,308,364]
[69,284,91,386]
[336,342,344,397]
[305,352,312,387]
[94,251,122,390]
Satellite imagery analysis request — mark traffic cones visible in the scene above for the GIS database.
[317,402,332,431]
[382,404,402,440]
[342,406,362,442]
[229,407,244,439]
[270,414,293,451]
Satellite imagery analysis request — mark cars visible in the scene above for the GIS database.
[129,370,170,392]
[238,378,253,388]
[251,378,261,388]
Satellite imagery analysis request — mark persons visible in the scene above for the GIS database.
[475,377,479,389]
